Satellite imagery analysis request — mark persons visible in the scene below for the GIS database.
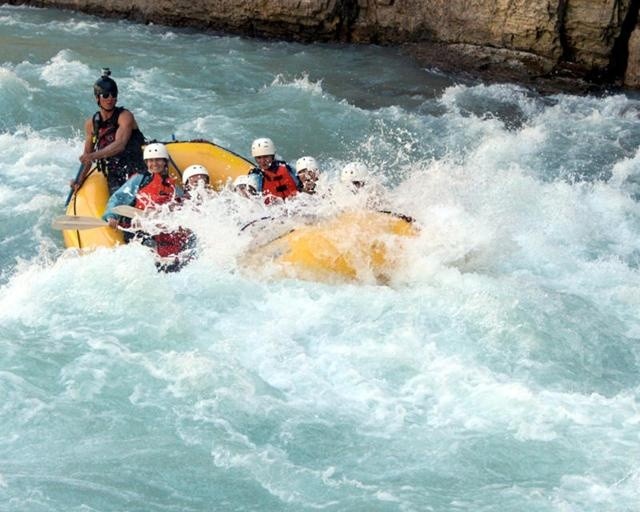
[228,173,260,202]
[338,158,373,193]
[290,153,332,197]
[69,76,151,240]
[246,135,301,206]
[172,164,217,210]
[102,140,188,255]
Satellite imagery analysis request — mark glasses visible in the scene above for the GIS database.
[99,92,117,98]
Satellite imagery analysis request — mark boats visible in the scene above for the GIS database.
[62,139,422,286]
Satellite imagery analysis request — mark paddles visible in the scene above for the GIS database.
[50,216,132,230]
[110,203,146,221]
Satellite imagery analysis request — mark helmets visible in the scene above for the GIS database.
[342,163,370,181]
[143,142,170,162]
[251,137,275,160]
[182,164,210,185]
[232,175,258,195]
[93,67,118,95]
[295,156,319,176]
